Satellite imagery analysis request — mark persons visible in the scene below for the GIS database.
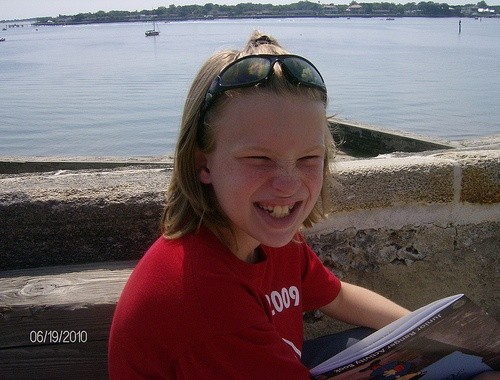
[109,30,500,380]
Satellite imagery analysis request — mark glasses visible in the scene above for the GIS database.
[193,55,328,147]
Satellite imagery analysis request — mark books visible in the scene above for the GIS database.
[310,294,500,380]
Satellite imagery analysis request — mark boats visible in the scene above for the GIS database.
[386,18,394,20]
[3,27,7,30]
[145,29,159,36]
[0,36,5,41]
